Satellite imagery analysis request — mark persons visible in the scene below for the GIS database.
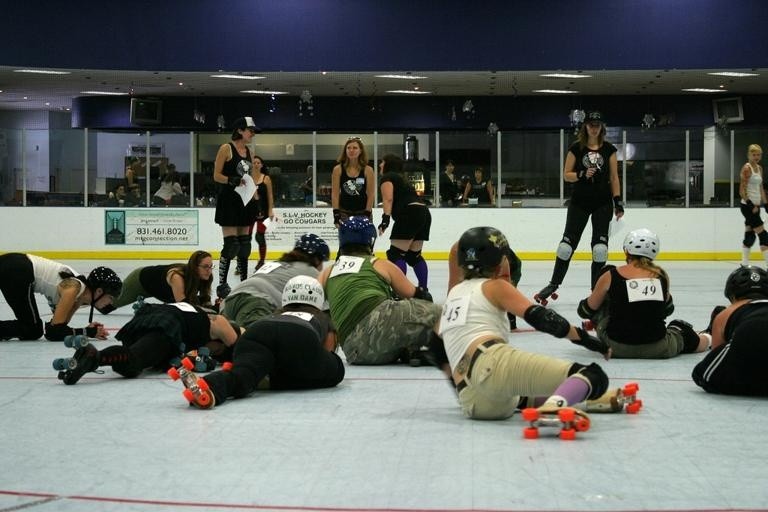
[740,144,768,264]
[332,137,375,226]
[0,248,121,340]
[541,112,624,298]
[300,166,313,204]
[447,228,521,329]
[234,155,274,275]
[325,216,453,383]
[441,161,495,204]
[378,155,427,283]
[580,227,724,358]
[437,227,608,418]
[693,266,766,396]
[100,160,189,205]
[54,235,345,406]
[214,117,259,298]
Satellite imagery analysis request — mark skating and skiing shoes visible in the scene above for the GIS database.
[215,284,231,304]
[172,344,215,372]
[587,384,642,413]
[53,335,99,385]
[522,402,590,440]
[167,358,215,409]
[234,260,265,273]
[221,361,269,397]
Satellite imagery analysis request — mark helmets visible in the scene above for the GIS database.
[339,214,377,248]
[282,275,324,310]
[458,226,510,265]
[624,228,659,260]
[724,265,767,299]
[88,266,121,299]
[295,234,329,260]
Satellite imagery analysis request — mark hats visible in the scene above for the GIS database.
[164,164,175,170]
[236,117,260,130]
[584,113,603,121]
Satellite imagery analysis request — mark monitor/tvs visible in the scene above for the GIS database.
[712,97,745,124]
[129,97,163,125]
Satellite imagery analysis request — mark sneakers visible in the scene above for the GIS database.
[534,283,559,306]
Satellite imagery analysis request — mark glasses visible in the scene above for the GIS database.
[197,264,215,270]
[348,137,362,140]
